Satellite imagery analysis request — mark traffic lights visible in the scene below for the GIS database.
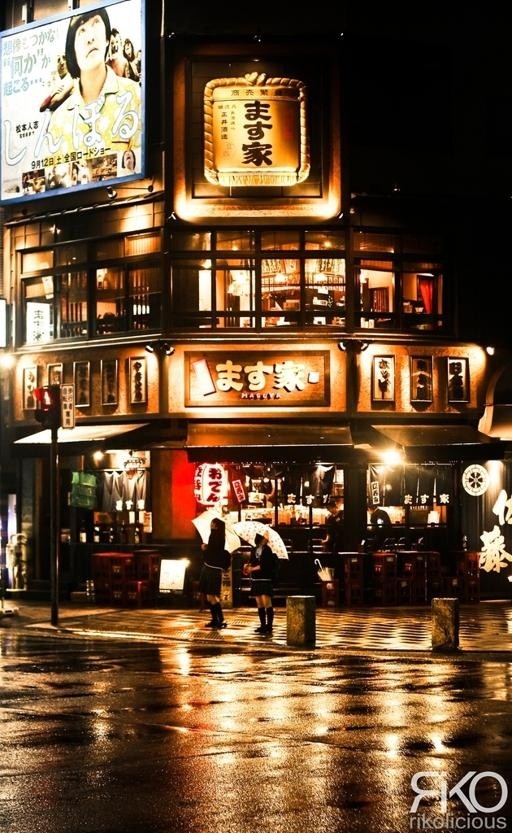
[31,383,63,433]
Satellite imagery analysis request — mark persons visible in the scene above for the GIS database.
[244,530,279,633]
[367,505,391,525]
[326,499,344,524]
[199,518,231,628]
[22,9,144,190]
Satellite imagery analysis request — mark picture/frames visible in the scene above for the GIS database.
[370,352,471,404]
[21,354,149,410]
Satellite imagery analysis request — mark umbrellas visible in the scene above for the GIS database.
[191,509,241,553]
[236,521,290,561]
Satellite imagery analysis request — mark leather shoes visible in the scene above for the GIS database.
[256,624,272,634]
[206,620,226,627]
